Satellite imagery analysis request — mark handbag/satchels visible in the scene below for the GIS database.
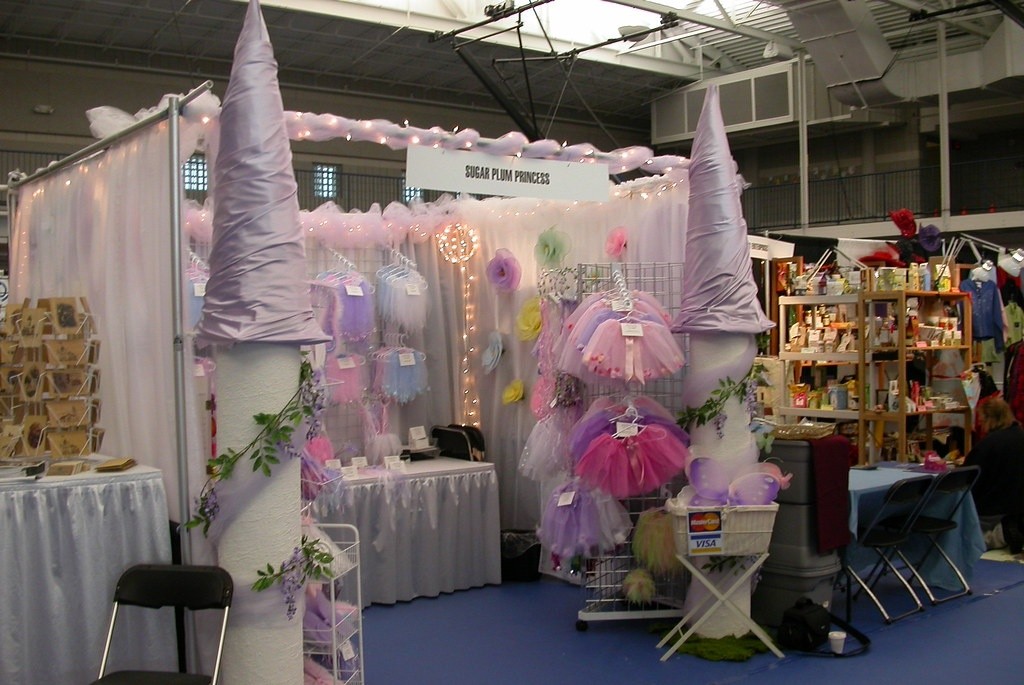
[776,597,871,657]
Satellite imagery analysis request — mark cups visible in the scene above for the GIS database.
[828,631,847,654]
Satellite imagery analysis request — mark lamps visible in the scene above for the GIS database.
[932,232,1024,287]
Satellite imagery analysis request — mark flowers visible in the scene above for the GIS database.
[279,426,302,459]
[295,369,334,442]
[265,545,321,619]
[483,251,520,295]
[606,227,631,253]
[482,330,508,376]
[497,379,526,405]
[516,303,546,344]
[531,228,570,269]
[192,478,221,528]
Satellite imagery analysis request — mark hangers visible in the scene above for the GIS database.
[606,387,669,444]
[602,269,649,325]
[321,247,428,367]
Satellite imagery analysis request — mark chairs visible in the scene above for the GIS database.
[838,467,983,623]
[92,565,233,685]
[430,424,486,462]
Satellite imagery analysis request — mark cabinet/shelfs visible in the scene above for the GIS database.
[300,523,364,684]
[779,289,890,462]
[854,290,972,465]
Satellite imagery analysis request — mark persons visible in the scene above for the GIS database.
[965,398,1024,555]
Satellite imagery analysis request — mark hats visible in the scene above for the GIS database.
[858,246,904,267]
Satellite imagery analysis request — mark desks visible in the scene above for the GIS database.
[322,455,502,607]
[843,468,986,589]
[0,454,181,685]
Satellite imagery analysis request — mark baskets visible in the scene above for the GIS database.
[768,421,836,440]
